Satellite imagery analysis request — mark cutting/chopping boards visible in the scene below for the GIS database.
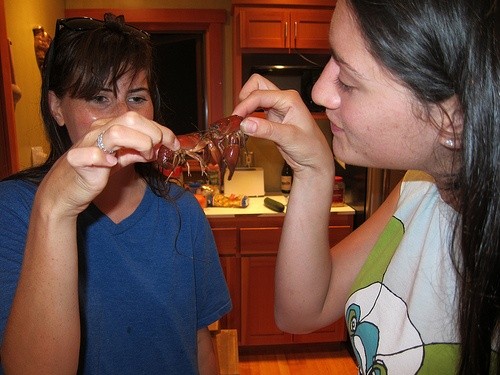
[202,195,288,216]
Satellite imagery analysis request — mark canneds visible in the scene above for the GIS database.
[331,177,345,208]
[241,151,255,167]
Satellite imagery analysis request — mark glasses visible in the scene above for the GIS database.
[49,15,151,82]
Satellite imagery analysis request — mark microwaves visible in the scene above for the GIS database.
[248,64,326,112]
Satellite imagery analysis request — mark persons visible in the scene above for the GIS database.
[232,0,500,375]
[0,12,232,375]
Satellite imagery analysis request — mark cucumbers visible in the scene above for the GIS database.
[264,197,284,212]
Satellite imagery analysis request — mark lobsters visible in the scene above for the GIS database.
[151,130,211,186]
[209,114,250,180]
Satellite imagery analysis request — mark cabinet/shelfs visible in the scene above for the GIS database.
[230,0,338,55]
[208,216,241,344]
[239,214,353,355]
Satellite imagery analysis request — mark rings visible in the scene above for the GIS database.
[98,133,114,154]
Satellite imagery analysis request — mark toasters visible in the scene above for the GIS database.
[223,167,266,197]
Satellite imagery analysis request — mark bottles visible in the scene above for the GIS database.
[331,176,345,206]
[280,161,292,194]
[205,163,221,191]
